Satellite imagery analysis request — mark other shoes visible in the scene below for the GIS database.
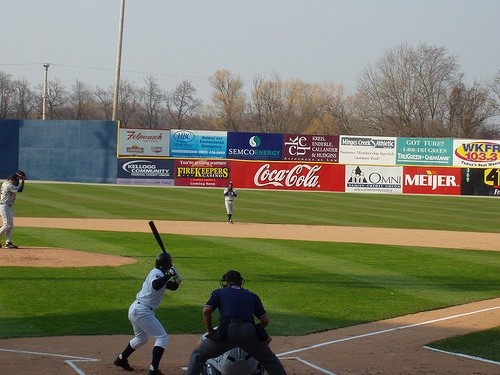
[229,220,233,223]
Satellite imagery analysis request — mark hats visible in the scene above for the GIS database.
[229,181,232,184]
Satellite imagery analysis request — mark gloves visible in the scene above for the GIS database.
[170,266,176,275]
[174,274,182,284]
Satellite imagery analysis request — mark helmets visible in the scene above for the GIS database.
[8,173,17,179]
[156,253,172,269]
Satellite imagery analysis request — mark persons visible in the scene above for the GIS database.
[0,173,25,249]
[198,325,272,375]
[114,252,182,375]
[224,182,238,224]
[184,270,287,375]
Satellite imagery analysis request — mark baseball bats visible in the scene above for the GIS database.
[148,220,182,285]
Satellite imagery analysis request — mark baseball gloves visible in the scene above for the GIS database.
[16,170,27,180]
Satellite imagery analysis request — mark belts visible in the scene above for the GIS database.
[227,354,251,362]
[1,203,11,206]
[233,319,252,323]
[138,302,153,310]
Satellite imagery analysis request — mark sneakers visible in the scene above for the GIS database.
[148,365,164,375]
[4,242,18,249]
[114,354,134,371]
